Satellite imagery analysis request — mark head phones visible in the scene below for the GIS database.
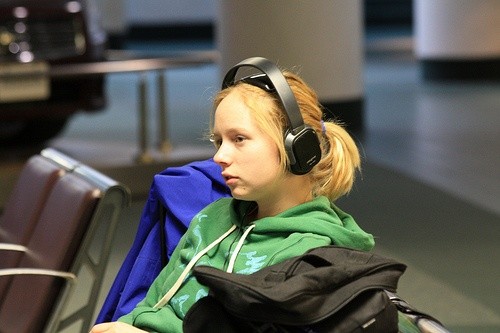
[222,55,322,175]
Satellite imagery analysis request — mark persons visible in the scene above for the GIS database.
[92,69,420,333]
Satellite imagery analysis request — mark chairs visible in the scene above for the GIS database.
[0,146,131,333]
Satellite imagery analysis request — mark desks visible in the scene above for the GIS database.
[0,50,215,163]
